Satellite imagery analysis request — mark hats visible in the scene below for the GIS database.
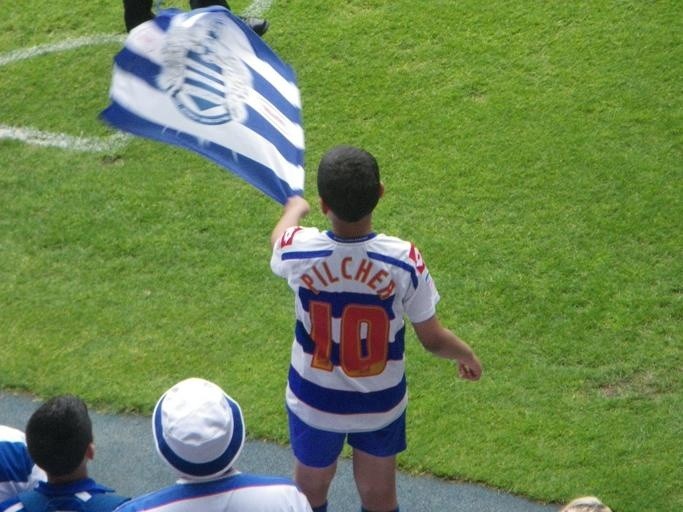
[151,377,246,480]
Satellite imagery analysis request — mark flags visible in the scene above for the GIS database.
[95,5,306,207]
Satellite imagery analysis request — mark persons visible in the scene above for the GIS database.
[107,377,314,510]
[118,1,273,39]
[269,144,484,510]
[0,423,47,504]
[0,394,133,512]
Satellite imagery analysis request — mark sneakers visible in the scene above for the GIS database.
[236,14,268,38]
